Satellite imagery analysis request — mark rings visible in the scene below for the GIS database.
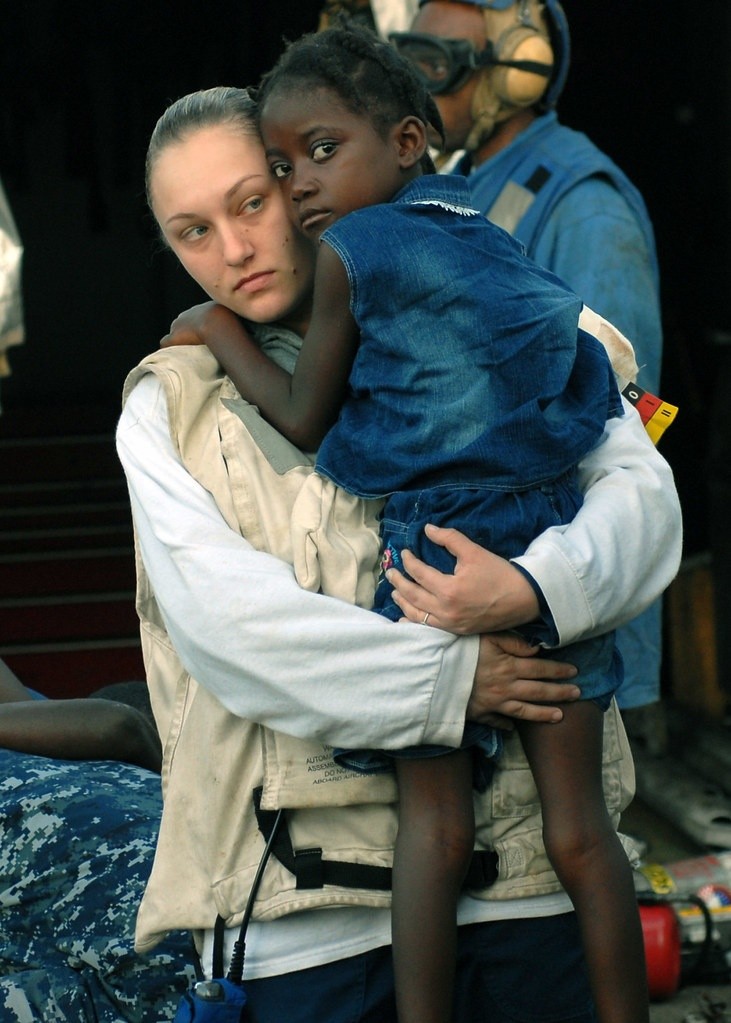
[422,613,430,626]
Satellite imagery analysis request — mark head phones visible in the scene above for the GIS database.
[489,1,555,107]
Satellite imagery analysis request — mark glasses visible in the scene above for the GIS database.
[387,30,484,96]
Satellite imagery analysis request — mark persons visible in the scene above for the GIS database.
[159,26,650,1023]
[389,0,660,707]
[0,663,203,1023]
[111,89,682,1023]
